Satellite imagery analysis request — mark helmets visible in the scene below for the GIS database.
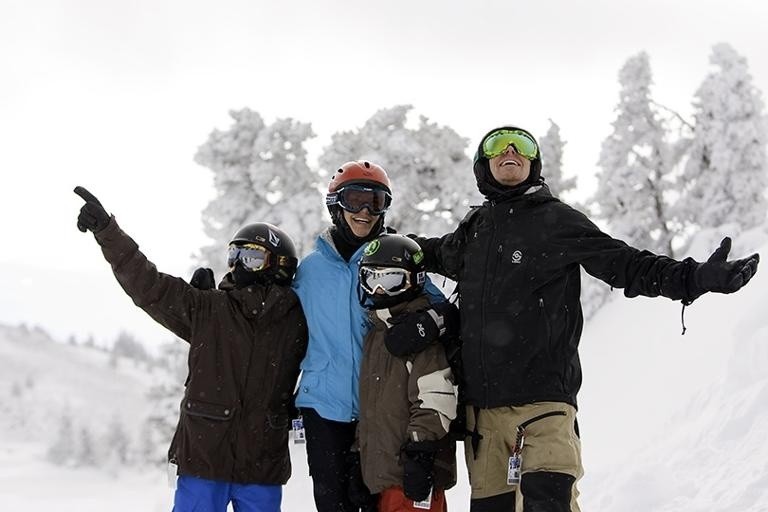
[357,233,428,309]
[326,160,394,219]
[227,222,298,285]
[473,126,540,185]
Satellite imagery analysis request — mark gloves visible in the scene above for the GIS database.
[190,267,217,291]
[382,302,458,358]
[72,183,111,234]
[697,236,761,295]
[400,441,440,501]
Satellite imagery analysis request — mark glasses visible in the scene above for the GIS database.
[475,128,539,167]
[358,265,414,296]
[227,242,297,274]
[326,185,392,217]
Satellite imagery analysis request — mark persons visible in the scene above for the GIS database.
[188,159,460,511]
[70,184,310,512]
[386,126,760,511]
[345,232,460,511]
